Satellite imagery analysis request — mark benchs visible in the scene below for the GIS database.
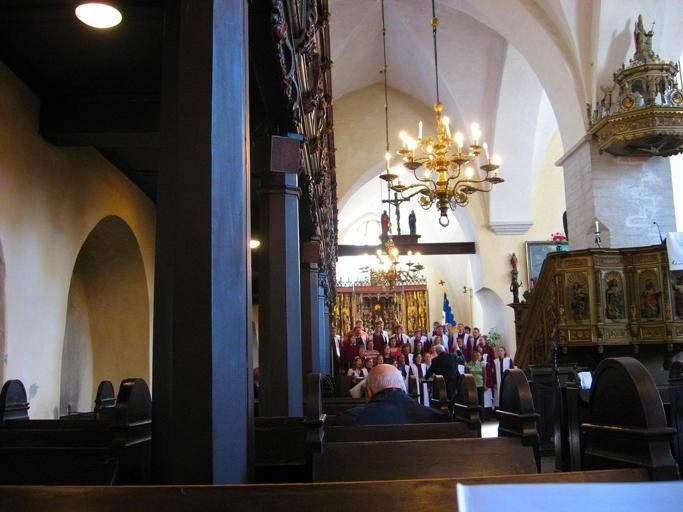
[319,393,537,482]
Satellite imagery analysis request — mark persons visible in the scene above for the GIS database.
[381,210,389,235]
[571,283,585,320]
[585,84,616,126]
[607,278,623,319]
[331,318,513,425]
[409,210,416,235]
[635,14,653,52]
[643,279,657,314]
[672,275,683,316]
[254,368,260,398]
[511,253,517,269]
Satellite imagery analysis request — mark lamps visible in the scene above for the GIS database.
[379,3,507,229]
